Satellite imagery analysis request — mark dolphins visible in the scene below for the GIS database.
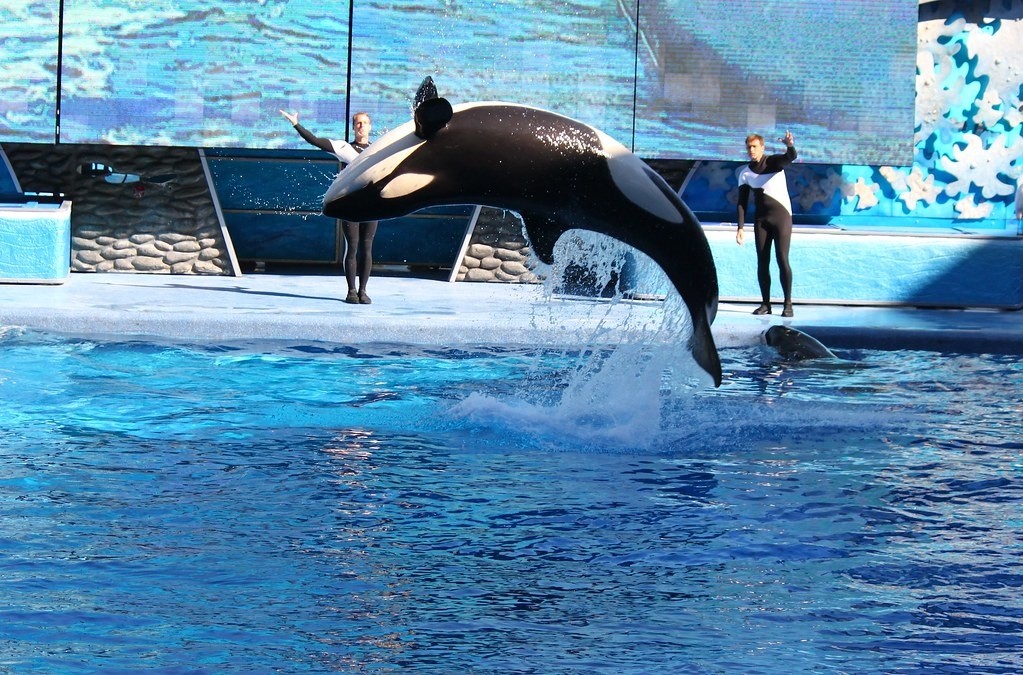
[760,324,837,361]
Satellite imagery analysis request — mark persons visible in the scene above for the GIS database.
[736,129,797,316]
[279,110,379,303]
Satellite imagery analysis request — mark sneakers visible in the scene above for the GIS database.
[357,290,371,303]
[753,303,771,314]
[783,304,793,316]
[346,289,359,304]
